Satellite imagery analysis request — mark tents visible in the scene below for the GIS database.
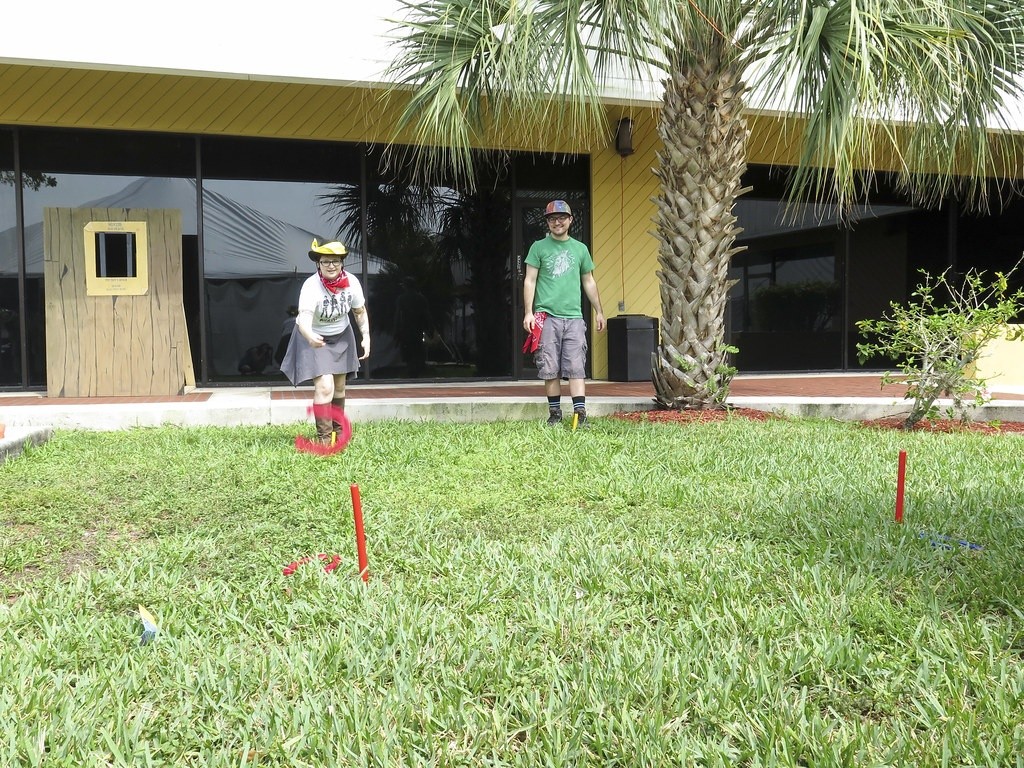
[0,178,403,382]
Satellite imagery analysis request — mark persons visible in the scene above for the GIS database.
[237,343,275,377]
[281,238,370,444]
[523,200,605,429]
[274,305,299,367]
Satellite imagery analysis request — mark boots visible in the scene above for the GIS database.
[332,397,346,437]
[314,402,333,447]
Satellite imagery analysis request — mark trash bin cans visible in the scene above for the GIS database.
[606,314,660,383]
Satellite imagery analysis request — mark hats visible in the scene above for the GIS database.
[308,238,349,261]
[543,200,571,218]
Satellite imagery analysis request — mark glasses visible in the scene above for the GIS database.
[319,260,342,266]
[548,216,569,222]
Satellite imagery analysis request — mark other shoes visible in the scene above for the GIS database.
[547,410,563,423]
[575,410,587,425]
